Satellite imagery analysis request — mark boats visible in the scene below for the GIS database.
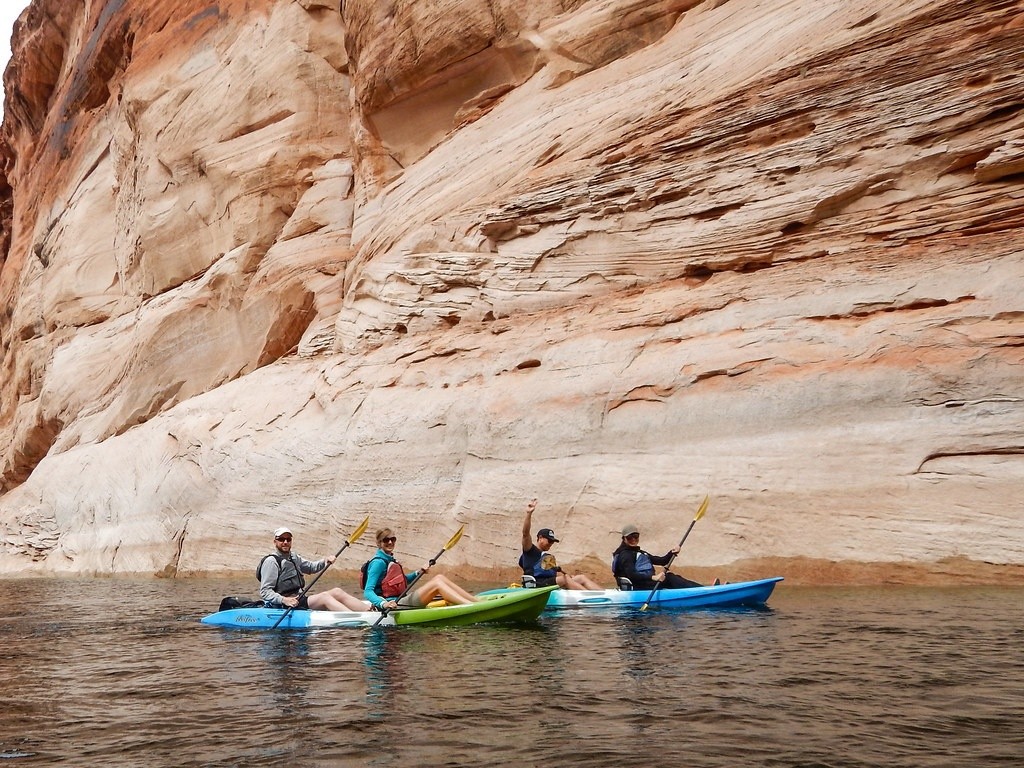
[471,575,783,612]
[198,579,560,633]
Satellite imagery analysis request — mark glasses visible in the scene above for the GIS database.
[276,537,293,542]
[543,538,554,544]
[624,533,639,541]
[379,537,397,543]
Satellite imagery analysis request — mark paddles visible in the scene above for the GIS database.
[541,555,567,576]
[641,494,709,611]
[271,516,369,629]
[372,525,465,626]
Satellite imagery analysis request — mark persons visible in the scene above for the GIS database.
[260,528,371,612]
[611,524,730,591]
[519,498,603,591]
[363,527,479,612]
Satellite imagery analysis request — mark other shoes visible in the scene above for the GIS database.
[712,577,720,586]
[724,581,730,584]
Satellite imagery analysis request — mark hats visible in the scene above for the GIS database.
[537,528,560,543]
[621,524,639,539]
[274,527,293,540]
